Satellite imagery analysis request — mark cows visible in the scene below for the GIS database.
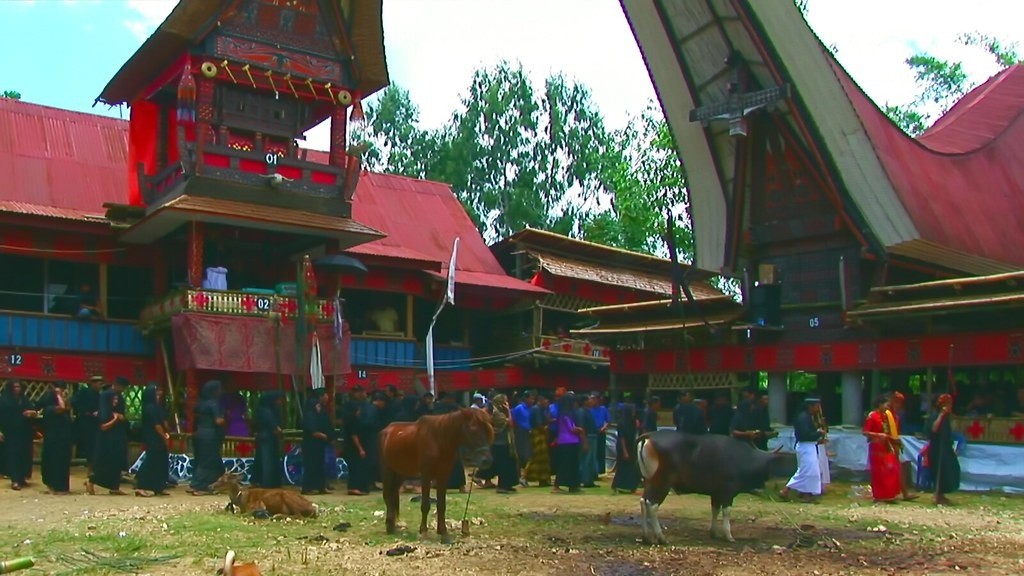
[633,430,798,540]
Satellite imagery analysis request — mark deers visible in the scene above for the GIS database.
[208,460,320,522]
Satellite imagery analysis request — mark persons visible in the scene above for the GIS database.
[75,280,102,317]
[0,374,779,496]
[777,394,831,503]
[370,300,400,332]
[863,388,920,503]
[925,393,960,507]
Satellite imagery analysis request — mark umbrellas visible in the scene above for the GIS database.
[313,255,369,278]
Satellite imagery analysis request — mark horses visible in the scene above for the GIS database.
[380,406,494,540]
